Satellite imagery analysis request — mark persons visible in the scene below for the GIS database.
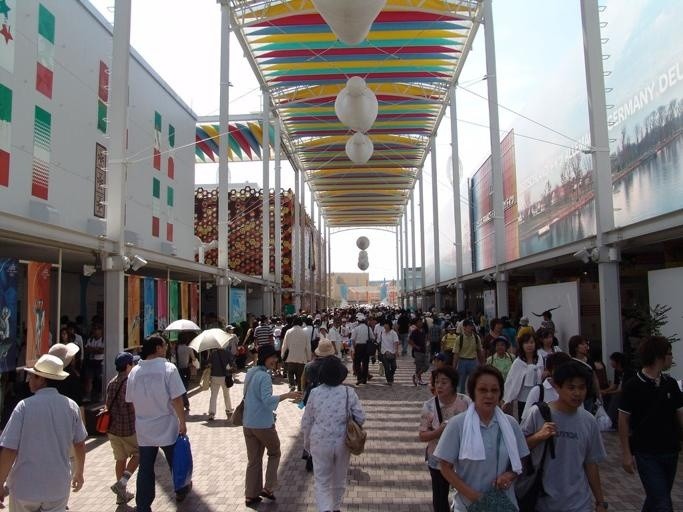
[0,295,682,512]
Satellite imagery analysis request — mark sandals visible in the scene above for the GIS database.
[245,496,262,507]
[260,487,276,501]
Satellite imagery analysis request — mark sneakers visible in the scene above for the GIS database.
[283,370,288,378]
[416,378,429,385]
[290,385,296,393]
[115,491,134,505]
[355,378,368,386]
[208,413,215,421]
[110,479,127,500]
[175,480,193,502]
[412,373,419,386]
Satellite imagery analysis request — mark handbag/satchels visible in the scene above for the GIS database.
[95,404,112,434]
[172,433,193,492]
[377,360,385,376]
[231,400,244,426]
[512,453,545,512]
[377,341,384,362]
[224,374,235,388]
[179,367,192,382]
[344,416,367,454]
[365,338,376,356]
[199,367,211,390]
[466,483,516,512]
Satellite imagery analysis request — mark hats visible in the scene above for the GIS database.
[256,344,282,366]
[425,311,451,320]
[273,328,282,337]
[22,353,70,381]
[314,337,335,357]
[359,316,366,321]
[341,318,347,322]
[226,324,236,331]
[114,352,141,367]
[519,316,529,326]
[47,341,80,370]
[491,335,511,350]
[317,353,348,387]
